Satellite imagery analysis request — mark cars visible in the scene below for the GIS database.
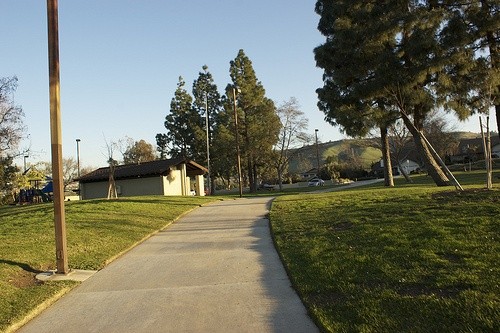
[308,178,325,187]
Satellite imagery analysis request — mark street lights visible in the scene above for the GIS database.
[24,155,29,172]
[315,129,319,177]
[76,139,80,195]
